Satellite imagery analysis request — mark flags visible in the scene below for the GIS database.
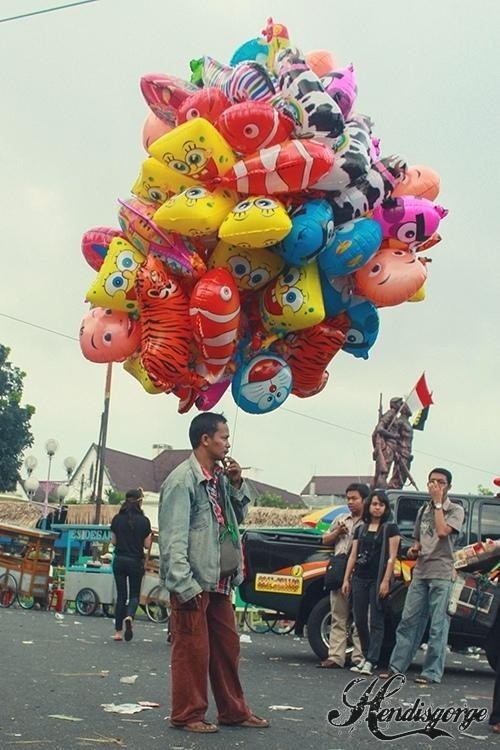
[408,390,433,430]
[408,371,434,417]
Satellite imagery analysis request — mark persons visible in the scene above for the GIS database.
[371,397,404,488]
[100,552,113,564]
[110,488,152,642]
[483,604,500,735]
[387,402,414,489]
[156,411,272,735]
[340,490,402,677]
[313,482,373,669]
[378,467,466,686]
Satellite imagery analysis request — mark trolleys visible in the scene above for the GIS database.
[0,522,299,634]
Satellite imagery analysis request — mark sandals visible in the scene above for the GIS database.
[315,660,340,668]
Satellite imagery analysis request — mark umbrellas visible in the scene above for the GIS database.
[300,503,352,533]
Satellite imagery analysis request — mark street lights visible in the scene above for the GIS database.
[25,438,77,530]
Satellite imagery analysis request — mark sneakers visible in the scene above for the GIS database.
[360,661,378,675]
[349,658,366,673]
[114,633,123,640]
[124,616,133,641]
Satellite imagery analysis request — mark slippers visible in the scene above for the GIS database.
[414,675,435,684]
[218,713,269,729]
[378,670,393,679]
[168,719,219,733]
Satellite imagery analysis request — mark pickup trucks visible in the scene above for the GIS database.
[239,490,500,666]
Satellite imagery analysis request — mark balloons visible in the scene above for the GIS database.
[74,11,453,416]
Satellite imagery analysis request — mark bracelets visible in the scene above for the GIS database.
[433,503,442,509]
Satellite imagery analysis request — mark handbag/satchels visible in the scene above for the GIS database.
[219,526,240,578]
[377,581,408,618]
[322,552,347,591]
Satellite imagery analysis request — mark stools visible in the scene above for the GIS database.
[48,589,64,613]
